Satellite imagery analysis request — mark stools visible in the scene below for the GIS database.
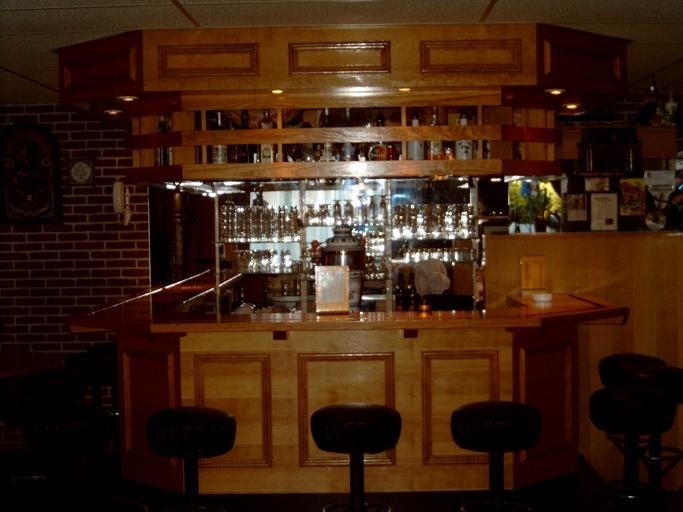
[451,400,541,512]
[588,389,677,512]
[598,353,683,512]
[309,404,402,512]
[141,408,236,512]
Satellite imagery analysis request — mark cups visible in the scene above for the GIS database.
[355,195,366,219]
[379,195,388,219]
[403,247,477,264]
[231,250,292,273]
[343,199,353,217]
[332,200,341,217]
[368,194,377,219]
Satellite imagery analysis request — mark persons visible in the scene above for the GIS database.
[665,169,683,233]
[509,176,564,231]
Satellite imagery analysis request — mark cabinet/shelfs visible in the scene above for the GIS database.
[303,181,391,313]
[130,99,562,180]
[391,176,483,311]
[216,195,302,315]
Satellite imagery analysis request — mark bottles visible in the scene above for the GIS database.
[341,107,356,161]
[424,106,444,160]
[210,110,228,164]
[321,107,336,160]
[257,109,276,162]
[367,111,398,161]
[238,109,257,162]
[455,114,472,161]
[407,110,425,160]
